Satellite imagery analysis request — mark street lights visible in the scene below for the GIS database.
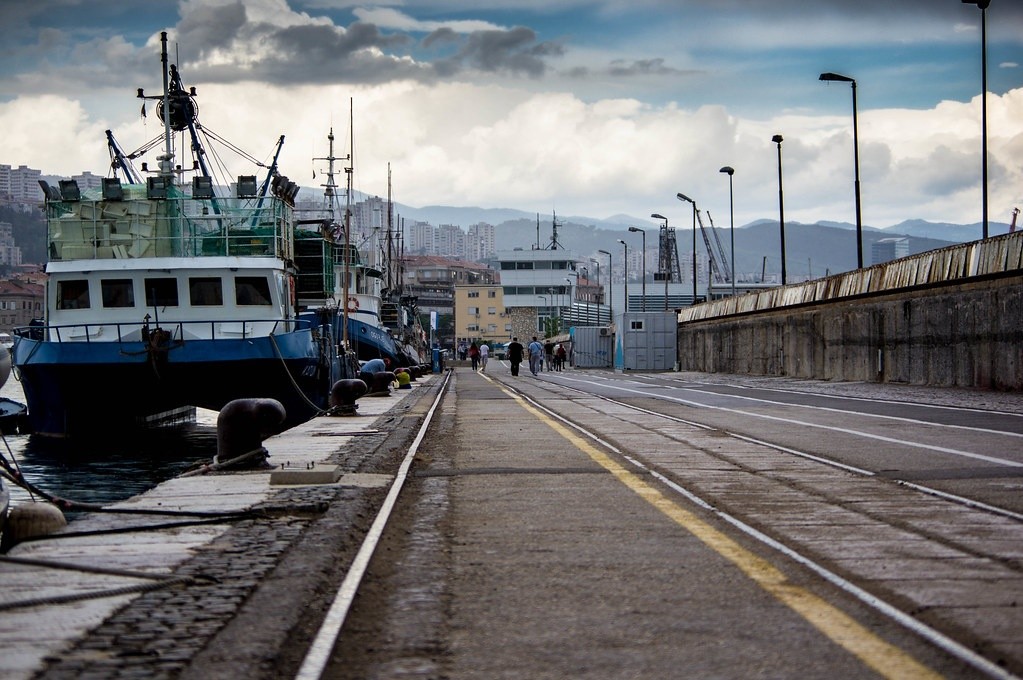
[771,134,786,286]
[566,279,571,327]
[650,213,668,313]
[719,165,735,295]
[616,238,627,312]
[548,288,559,306]
[819,72,862,270]
[598,249,613,324]
[589,258,600,326]
[629,227,645,312]
[567,271,579,327]
[677,192,697,306]
[580,266,588,326]
[962,0,990,239]
[538,295,547,317]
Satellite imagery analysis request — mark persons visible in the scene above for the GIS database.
[506,337,524,377]
[360,358,391,393]
[528,337,545,376]
[543,340,554,373]
[552,340,567,372]
[458,343,468,360]
[479,340,490,372]
[452,346,456,361]
[469,342,480,371]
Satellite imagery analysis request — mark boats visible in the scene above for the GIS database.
[8,29,428,463]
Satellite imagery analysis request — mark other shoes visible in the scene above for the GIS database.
[559,369,561,371]
[562,367,565,369]
[550,369,553,371]
[534,373,537,376]
[556,369,558,371]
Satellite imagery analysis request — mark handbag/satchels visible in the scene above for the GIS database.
[397,370,412,389]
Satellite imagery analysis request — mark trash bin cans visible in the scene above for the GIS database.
[431,350,443,372]
